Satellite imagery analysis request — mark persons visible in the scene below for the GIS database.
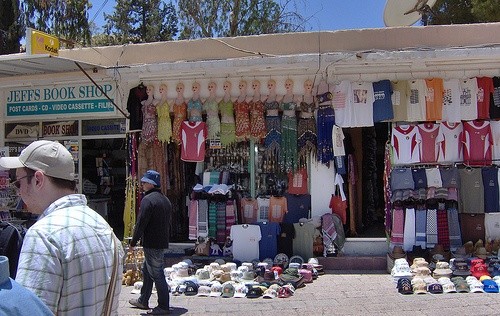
[0,256,54,316]
[129,170,172,314]
[314,80,335,163]
[0,140,124,316]
[218,81,236,144]
[155,85,172,141]
[297,80,315,161]
[141,86,156,143]
[282,80,297,173]
[187,82,204,121]
[171,84,188,141]
[204,82,220,136]
[233,82,250,136]
[263,79,281,172]
[249,80,266,136]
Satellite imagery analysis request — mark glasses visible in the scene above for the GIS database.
[9,174,28,190]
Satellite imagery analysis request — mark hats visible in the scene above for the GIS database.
[386,244,500,294]
[0,140,79,181]
[141,170,161,188]
[130,258,325,299]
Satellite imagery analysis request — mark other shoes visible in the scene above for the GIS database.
[129,299,149,309]
[146,306,169,314]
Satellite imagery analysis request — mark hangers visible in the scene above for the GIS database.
[465,160,473,170]
[356,73,363,85]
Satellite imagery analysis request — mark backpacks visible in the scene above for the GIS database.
[0,220,28,280]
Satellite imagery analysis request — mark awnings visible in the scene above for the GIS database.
[0,54,129,119]
